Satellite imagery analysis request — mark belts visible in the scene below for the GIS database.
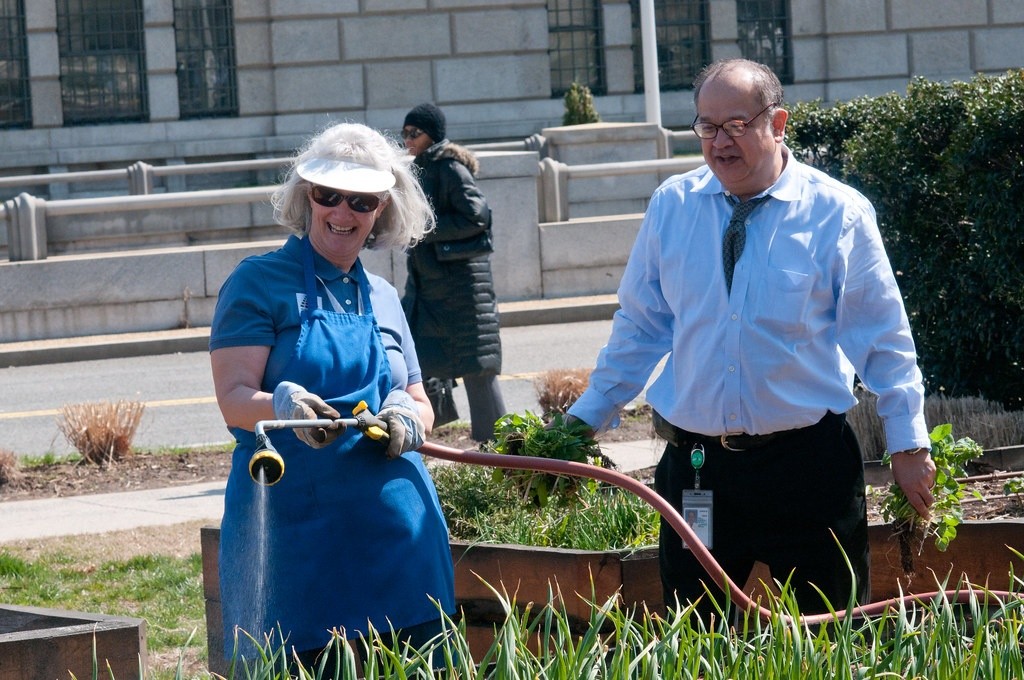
[647,409,816,458]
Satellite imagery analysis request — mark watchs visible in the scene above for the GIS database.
[891,447,921,456]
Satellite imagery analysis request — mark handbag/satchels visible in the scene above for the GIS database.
[432,208,493,261]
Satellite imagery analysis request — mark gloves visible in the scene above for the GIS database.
[374,390,426,460]
[273,381,347,450]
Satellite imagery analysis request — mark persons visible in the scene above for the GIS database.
[544,60,937,643]
[402,105,509,439]
[686,511,697,530]
[208,123,458,680]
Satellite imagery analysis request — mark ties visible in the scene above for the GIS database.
[720,192,764,296]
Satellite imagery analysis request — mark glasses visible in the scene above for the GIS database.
[310,184,379,212]
[398,128,422,138]
[691,101,778,140]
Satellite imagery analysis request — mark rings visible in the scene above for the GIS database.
[929,481,936,490]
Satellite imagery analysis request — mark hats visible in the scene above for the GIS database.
[294,147,397,193]
[401,103,446,142]
